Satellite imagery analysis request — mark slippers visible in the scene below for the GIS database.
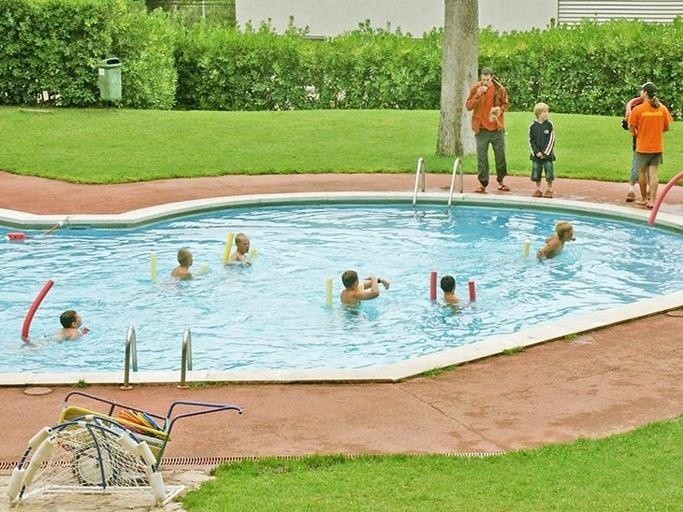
[498,184,510,191]
[473,186,486,194]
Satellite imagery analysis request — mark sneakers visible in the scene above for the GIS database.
[625,189,656,209]
[532,189,554,198]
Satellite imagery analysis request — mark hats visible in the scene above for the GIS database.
[635,80,658,93]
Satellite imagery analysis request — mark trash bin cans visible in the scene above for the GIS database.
[96,57,122,112]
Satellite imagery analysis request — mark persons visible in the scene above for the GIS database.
[465,66,510,194]
[231,233,250,264]
[341,270,390,306]
[536,221,576,259]
[171,249,194,279]
[621,96,646,202]
[627,82,671,208]
[441,275,459,304]
[56,310,90,338]
[528,102,556,198]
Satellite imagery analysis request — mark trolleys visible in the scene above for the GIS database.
[56,391,244,486]
